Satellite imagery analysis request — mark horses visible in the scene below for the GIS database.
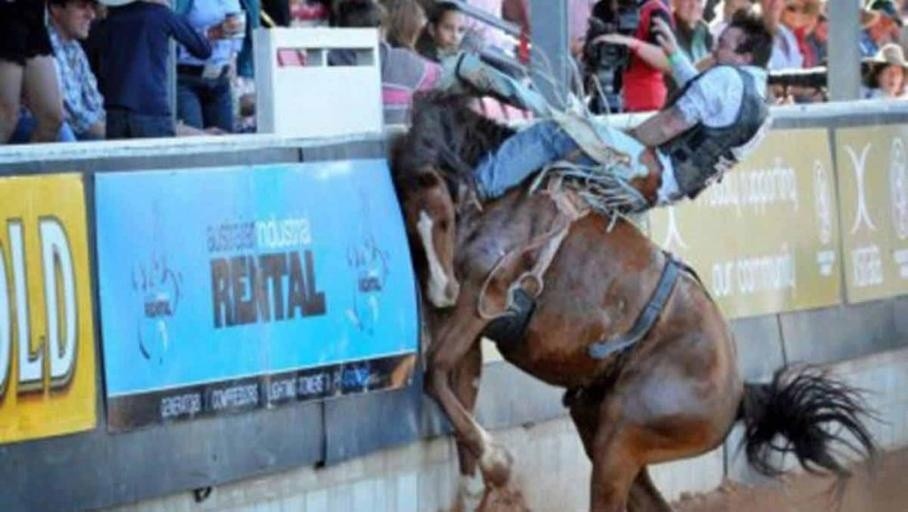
[387,87,897,512]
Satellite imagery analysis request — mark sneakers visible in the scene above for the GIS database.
[443,159,484,221]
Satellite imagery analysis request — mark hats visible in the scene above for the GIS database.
[858,45,908,77]
[871,3,904,25]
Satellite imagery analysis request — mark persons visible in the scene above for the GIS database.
[862,44,908,99]
[386,1,428,51]
[443,9,775,215]
[428,2,465,62]
[668,0,714,61]
[42,0,108,141]
[769,1,829,106]
[862,1,908,53]
[83,0,214,138]
[176,1,247,132]
[325,0,441,128]
[573,0,678,114]
[0,0,67,145]
